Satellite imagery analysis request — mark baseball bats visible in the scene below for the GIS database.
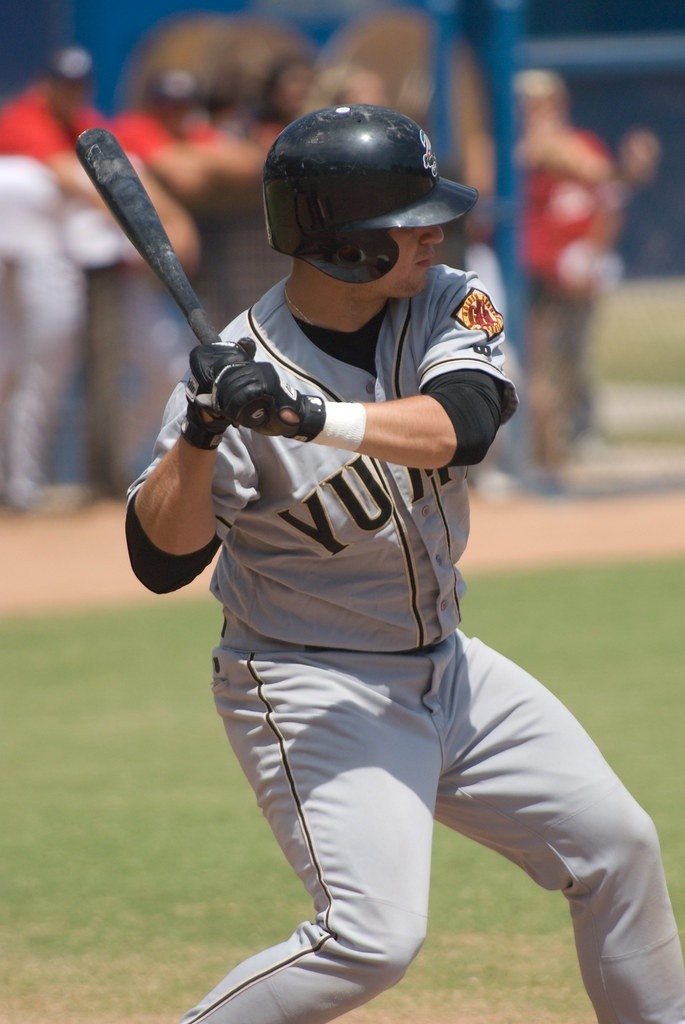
[75,126,276,429]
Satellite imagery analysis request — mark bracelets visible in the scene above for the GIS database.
[310,402,367,451]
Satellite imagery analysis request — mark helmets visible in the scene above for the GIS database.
[262,104,479,283]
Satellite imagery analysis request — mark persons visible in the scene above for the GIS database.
[0,42,661,506]
[122,102,685,1024]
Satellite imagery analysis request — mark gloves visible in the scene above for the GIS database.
[180,337,257,450]
[196,360,326,442]
